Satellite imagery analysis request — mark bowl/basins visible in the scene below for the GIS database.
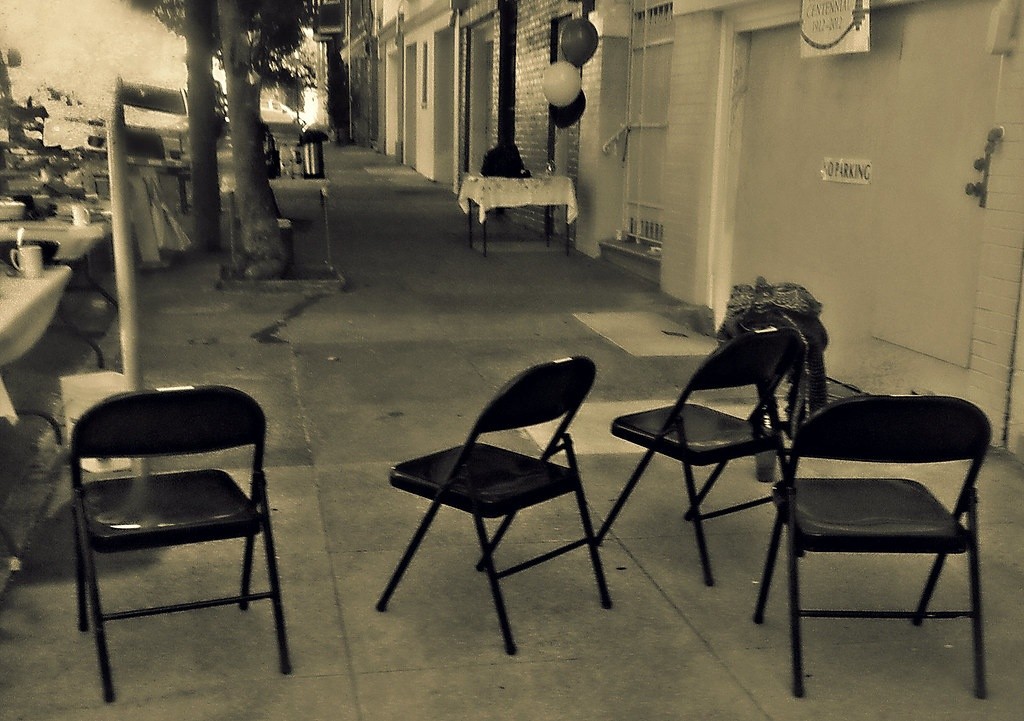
[0,200,26,218]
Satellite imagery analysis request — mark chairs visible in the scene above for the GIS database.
[753,394,994,699]
[69,385,290,704]
[594,326,812,585]
[375,354,612,655]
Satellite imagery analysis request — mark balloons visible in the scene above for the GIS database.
[548,88,586,129]
[543,62,581,106]
[561,18,598,68]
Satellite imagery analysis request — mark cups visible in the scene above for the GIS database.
[10,246,43,278]
[72,205,90,226]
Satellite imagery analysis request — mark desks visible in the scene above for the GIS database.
[0,195,113,223]
[0,221,119,369]
[459,173,579,257]
[0,266,75,574]
